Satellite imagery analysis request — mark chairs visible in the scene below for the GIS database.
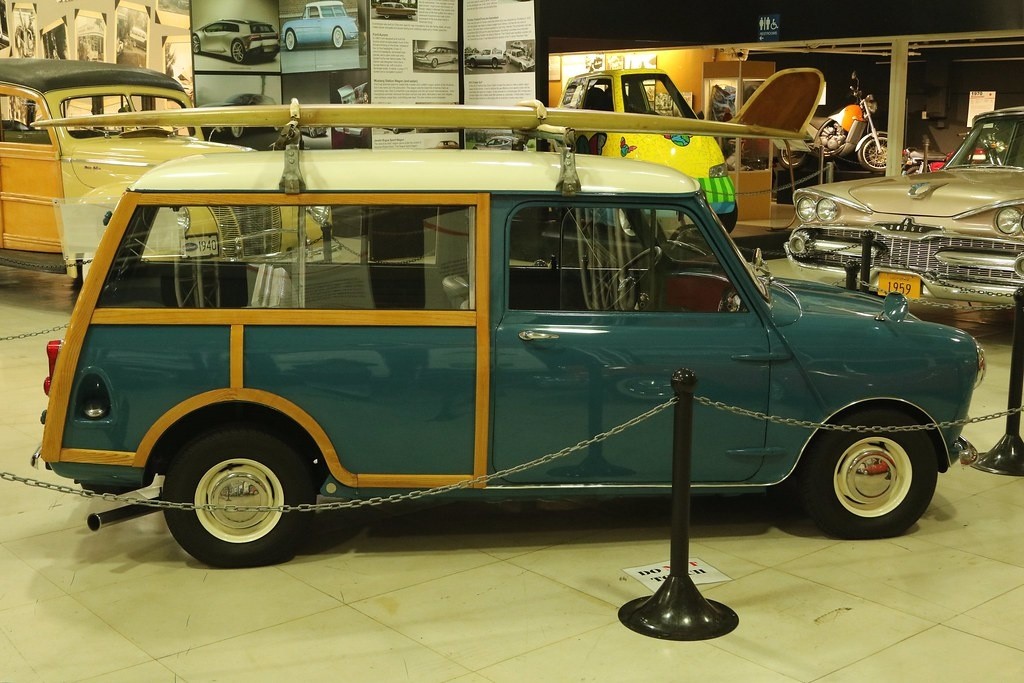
[246,263,293,312]
[440,277,469,310]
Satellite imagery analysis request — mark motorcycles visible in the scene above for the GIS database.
[778,71,887,172]
[901,124,1009,176]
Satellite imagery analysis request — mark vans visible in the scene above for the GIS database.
[0,57,333,298]
[129,26,146,48]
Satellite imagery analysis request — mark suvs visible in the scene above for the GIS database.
[192,19,280,64]
[465,49,503,68]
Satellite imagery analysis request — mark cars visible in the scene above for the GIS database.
[280,1,358,49]
[414,47,458,68]
[473,136,528,151]
[31,67,986,568]
[506,42,535,71]
[783,106,1024,338]
[549,68,739,244]
[376,2,417,20]
